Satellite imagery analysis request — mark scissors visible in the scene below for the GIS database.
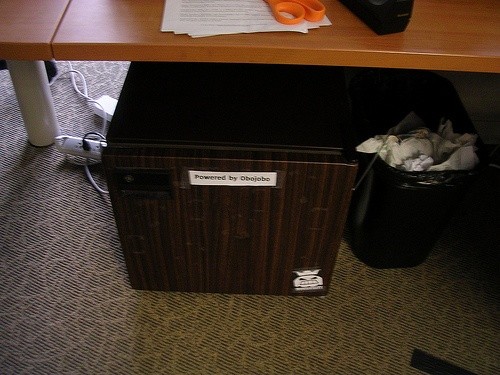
[263,0,326,25]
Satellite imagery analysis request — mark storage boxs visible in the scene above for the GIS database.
[100,60,363,296]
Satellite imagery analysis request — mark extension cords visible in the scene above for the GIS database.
[62,137,107,160]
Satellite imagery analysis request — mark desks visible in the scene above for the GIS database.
[0,0,499,149]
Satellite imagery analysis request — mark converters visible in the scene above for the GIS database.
[94,95,118,122]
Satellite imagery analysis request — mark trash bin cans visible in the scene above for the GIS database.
[346,121,479,269]
